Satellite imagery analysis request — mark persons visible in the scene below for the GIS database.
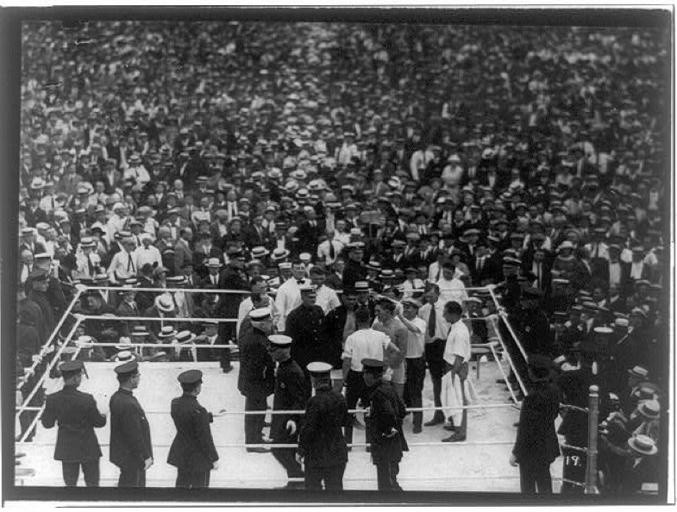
[557,360,666,492]
[285,266,342,316]
[341,307,401,424]
[441,301,471,443]
[295,362,353,491]
[267,335,310,489]
[236,277,281,409]
[361,358,409,492]
[284,285,325,397]
[393,298,426,433]
[510,353,561,493]
[417,283,451,426]
[16,24,665,362]
[109,361,154,487]
[167,369,227,488]
[496,271,550,403]
[275,259,312,335]
[238,292,275,437]
[215,249,250,373]
[17,356,63,465]
[41,361,106,487]
[237,308,275,453]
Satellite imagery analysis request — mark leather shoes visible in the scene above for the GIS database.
[413,417,466,443]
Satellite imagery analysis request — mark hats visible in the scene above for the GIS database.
[177,369,203,388]
[523,292,663,457]
[305,359,334,378]
[360,357,385,374]
[58,359,84,376]
[114,360,138,379]
